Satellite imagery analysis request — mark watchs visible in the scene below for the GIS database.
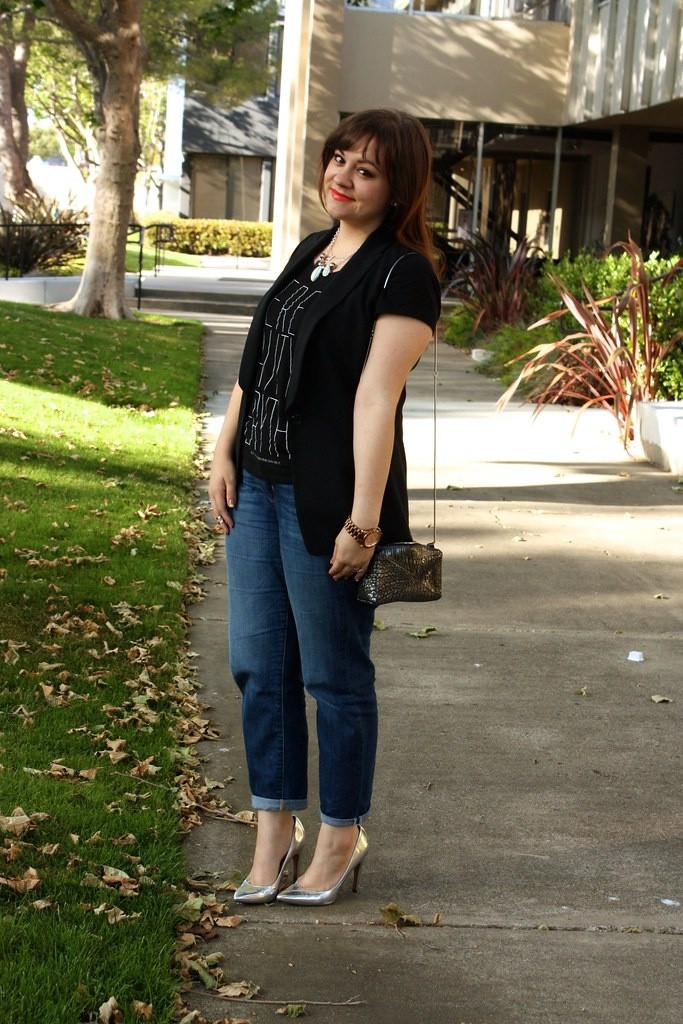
[345,517,383,547]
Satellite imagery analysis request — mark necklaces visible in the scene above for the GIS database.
[309,228,360,282]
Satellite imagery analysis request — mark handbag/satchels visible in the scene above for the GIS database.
[357,542,443,605]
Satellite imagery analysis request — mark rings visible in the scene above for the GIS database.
[356,569,363,572]
[216,516,224,525]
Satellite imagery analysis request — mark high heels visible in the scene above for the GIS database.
[233,817,306,902]
[277,824,369,905]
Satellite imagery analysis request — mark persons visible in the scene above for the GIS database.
[210,109,439,906]
